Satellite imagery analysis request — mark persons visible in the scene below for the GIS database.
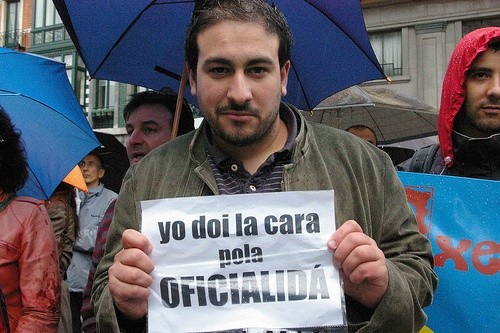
[395,27,500,180]
[0,87,381,333]
[80,0,437,332]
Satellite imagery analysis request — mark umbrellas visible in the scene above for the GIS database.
[0,0,440,203]
